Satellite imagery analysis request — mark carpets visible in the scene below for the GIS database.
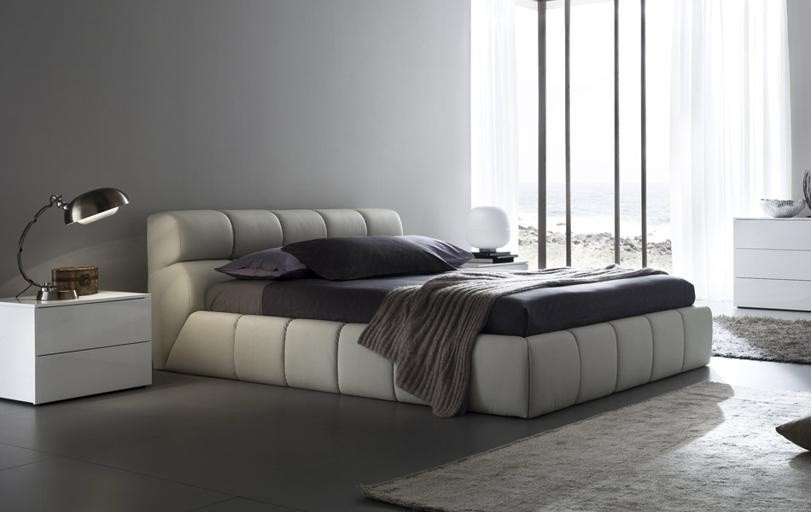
[713,314,811,364]
[361,380,811,511]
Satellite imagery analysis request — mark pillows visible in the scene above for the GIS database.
[388,233,474,267]
[215,239,306,282]
[778,414,811,452]
[291,234,458,282]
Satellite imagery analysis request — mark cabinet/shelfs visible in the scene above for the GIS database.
[731,217,811,313]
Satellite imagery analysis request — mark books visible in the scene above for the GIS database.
[469,252,519,263]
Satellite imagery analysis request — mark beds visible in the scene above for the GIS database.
[145,206,714,419]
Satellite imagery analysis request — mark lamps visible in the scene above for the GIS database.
[16,186,130,300]
[462,206,518,262]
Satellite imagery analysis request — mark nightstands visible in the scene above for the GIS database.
[0,290,152,405]
[462,261,529,270]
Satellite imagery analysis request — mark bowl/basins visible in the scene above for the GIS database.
[758,198,808,218]
